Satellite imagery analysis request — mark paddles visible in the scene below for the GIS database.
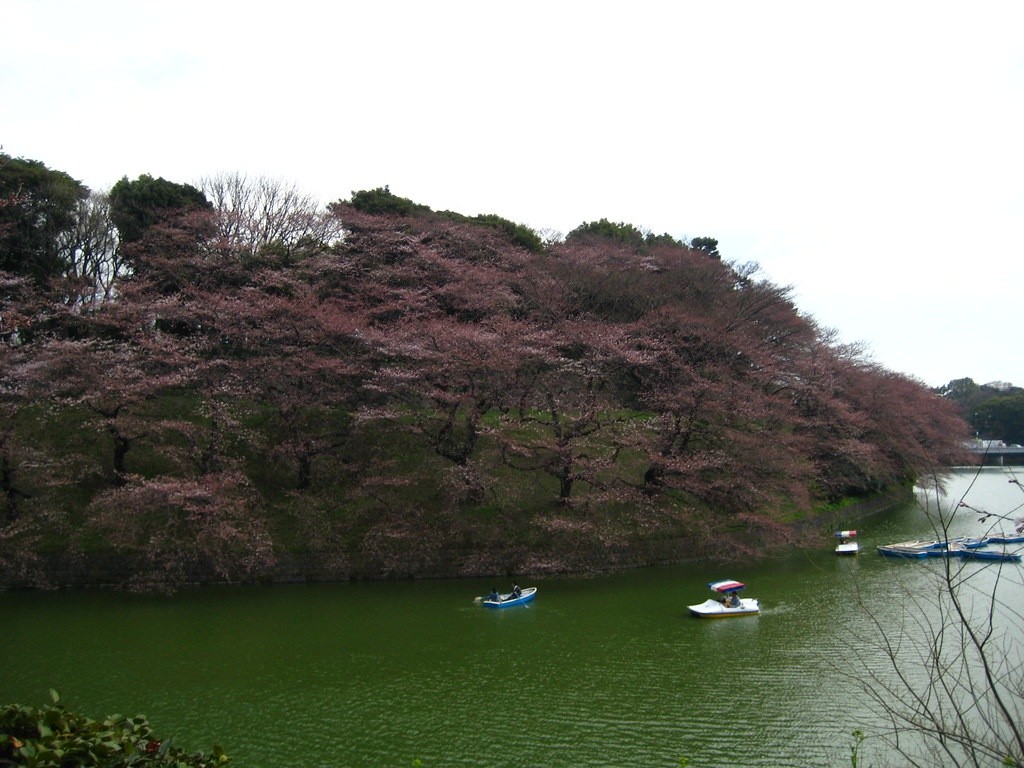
[514,591,530,608]
[475,597,489,601]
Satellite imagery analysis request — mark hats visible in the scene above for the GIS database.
[731,591,737,595]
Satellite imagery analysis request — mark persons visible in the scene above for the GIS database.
[730,592,740,608]
[488,588,501,601]
[722,598,730,608]
[507,582,522,600]
[840,538,845,544]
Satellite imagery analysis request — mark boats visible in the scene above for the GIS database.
[834,530,859,554]
[875,531,1024,563]
[685,580,761,619]
[483,587,538,609]
